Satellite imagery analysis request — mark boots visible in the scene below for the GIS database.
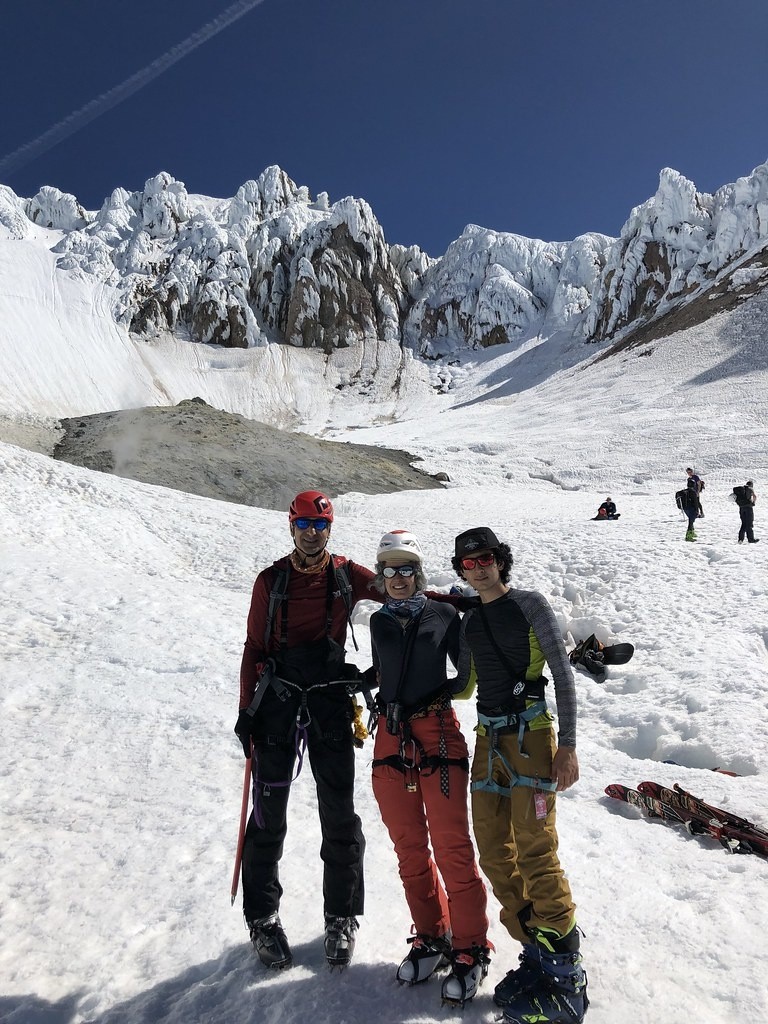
[692,528,697,537]
[686,529,697,542]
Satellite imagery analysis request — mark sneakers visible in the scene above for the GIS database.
[396,929,452,988]
[247,910,293,969]
[493,944,541,1007]
[502,948,590,1024]
[323,911,359,974]
[440,944,491,1009]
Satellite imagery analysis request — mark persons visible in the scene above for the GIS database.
[683,467,705,542]
[234,492,589,1024]
[737,481,760,544]
[598,497,621,520]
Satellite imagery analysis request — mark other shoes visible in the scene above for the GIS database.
[750,539,759,543]
[699,514,704,517]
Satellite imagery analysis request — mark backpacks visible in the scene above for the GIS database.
[597,508,608,520]
[733,485,752,506]
[695,475,705,492]
[676,489,694,510]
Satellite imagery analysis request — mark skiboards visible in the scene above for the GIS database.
[638,781,768,840]
[605,784,767,856]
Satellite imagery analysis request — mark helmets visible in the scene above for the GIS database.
[376,530,424,568]
[288,491,333,523]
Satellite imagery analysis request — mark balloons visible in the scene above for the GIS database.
[600,508,606,515]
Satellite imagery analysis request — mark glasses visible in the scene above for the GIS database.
[461,553,496,570]
[381,565,415,578]
[292,517,330,531]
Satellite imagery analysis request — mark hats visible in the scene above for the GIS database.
[606,497,611,501]
[454,527,500,559]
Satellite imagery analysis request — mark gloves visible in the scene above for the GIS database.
[458,595,482,613]
[235,709,257,759]
